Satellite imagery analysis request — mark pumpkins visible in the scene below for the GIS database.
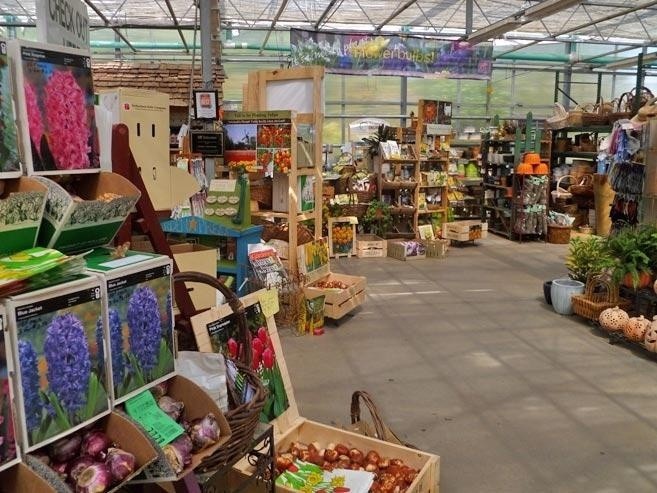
[626,271,650,288]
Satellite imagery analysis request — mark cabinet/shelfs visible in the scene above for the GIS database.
[221,109,323,315]
[160,216,265,298]
[363,96,657,243]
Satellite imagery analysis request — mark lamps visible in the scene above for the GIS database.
[322,144,333,170]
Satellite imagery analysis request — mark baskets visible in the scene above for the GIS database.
[340,181,375,222]
[570,272,634,324]
[551,175,593,203]
[252,218,315,246]
[174,271,269,476]
[546,85,655,129]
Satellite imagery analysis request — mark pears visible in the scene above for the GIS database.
[332,226,354,246]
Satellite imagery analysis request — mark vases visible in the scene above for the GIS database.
[551,279,586,316]
[544,281,552,305]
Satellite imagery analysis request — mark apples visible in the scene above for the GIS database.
[312,279,349,289]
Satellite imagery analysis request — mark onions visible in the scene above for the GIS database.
[151,382,221,476]
[36,430,138,493]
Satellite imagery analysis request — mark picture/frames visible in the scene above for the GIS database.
[189,288,302,450]
[328,216,357,258]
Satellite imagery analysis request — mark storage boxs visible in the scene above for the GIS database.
[355,233,387,259]
[303,272,366,318]
[387,239,428,262]
[422,238,448,259]
[0,35,230,493]
[231,416,440,493]
